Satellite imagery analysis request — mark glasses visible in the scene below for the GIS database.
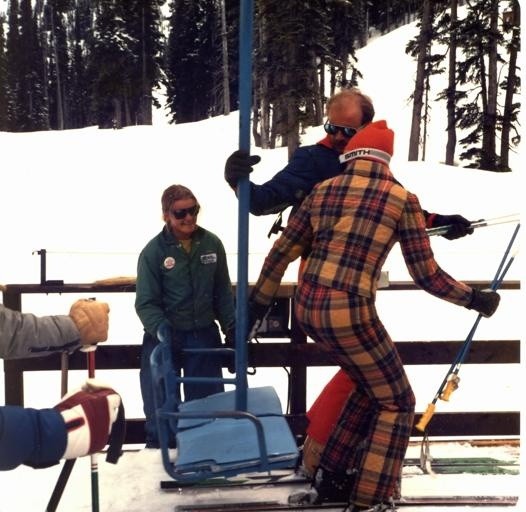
[322,116,370,138]
[167,200,202,220]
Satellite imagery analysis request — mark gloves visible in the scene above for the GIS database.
[422,209,475,241]
[463,286,501,319]
[50,380,124,462]
[68,297,112,347]
[223,146,262,193]
[155,317,184,351]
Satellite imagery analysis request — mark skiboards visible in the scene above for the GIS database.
[173,496,519,510]
[160,456,520,476]
[160,456,520,511]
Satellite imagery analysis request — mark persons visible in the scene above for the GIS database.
[1,384,121,472]
[135,184,235,448]
[1,299,109,361]
[225,90,474,476]
[247,121,500,512]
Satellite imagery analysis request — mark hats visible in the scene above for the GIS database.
[337,117,396,165]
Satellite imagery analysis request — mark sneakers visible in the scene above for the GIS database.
[309,462,360,506]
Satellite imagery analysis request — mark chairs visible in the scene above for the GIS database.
[147,345,300,483]
[151,340,285,432]
